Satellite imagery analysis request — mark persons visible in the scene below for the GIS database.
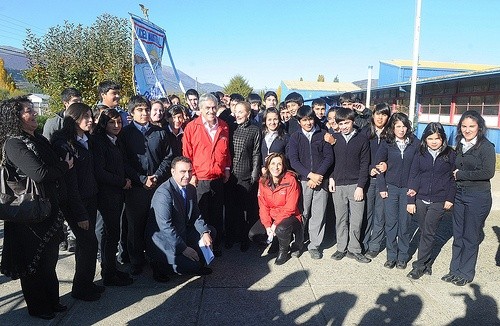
[407,122,457,278]
[288,106,333,259]
[144,157,216,282]
[43,82,391,257]
[248,152,301,265]
[120,96,176,279]
[224,101,261,249]
[441,110,496,286]
[1,98,73,320]
[328,109,370,263]
[92,109,134,285]
[376,113,421,269]
[182,94,231,257]
[51,103,105,301]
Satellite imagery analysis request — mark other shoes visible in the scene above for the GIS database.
[407,267,425,279]
[27,310,56,319]
[307,248,322,258]
[384,260,396,268]
[240,241,248,252]
[81,285,105,301]
[331,250,347,260]
[51,301,65,312]
[366,250,381,258]
[347,250,371,263]
[104,277,132,286]
[101,271,131,280]
[134,263,143,275]
[396,260,407,269]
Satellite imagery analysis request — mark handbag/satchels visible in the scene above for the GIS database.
[0,176,52,224]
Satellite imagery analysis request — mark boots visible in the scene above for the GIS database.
[275,225,292,264]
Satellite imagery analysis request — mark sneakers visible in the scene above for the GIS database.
[441,271,455,282]
[451,275,473,286]
